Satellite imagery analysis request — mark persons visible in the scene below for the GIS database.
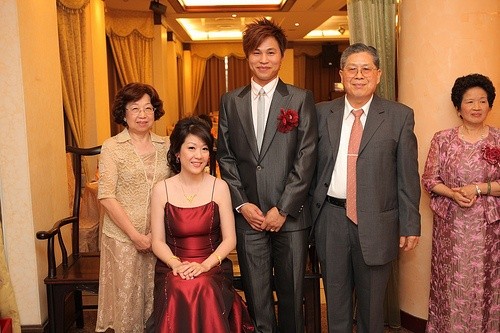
[422,73,500,333]
[95,83,174,333]
[313,44,421,333]
[150,116,237,333]
[217,19,318,333]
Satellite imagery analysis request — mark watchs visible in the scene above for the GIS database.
[277,208,288,216]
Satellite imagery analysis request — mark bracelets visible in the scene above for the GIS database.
[474,184,481,198]
[167,256,181,269]
[487,182,491,195]
[210,252,222,266]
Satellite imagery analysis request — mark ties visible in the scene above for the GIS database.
[346,109,364,225]
[257,89,265,154]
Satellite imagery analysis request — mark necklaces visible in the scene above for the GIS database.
[460,124,485,149]
[179,172,204,204]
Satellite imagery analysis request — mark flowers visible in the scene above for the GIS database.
[481,142,500,165]
[276,107,299,133]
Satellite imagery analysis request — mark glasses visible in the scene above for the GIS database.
[342,66,378,74]
[126,105,156,113]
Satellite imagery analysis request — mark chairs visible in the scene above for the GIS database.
[36,146,102,333]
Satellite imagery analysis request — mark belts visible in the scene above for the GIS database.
[325,196,347,207]
